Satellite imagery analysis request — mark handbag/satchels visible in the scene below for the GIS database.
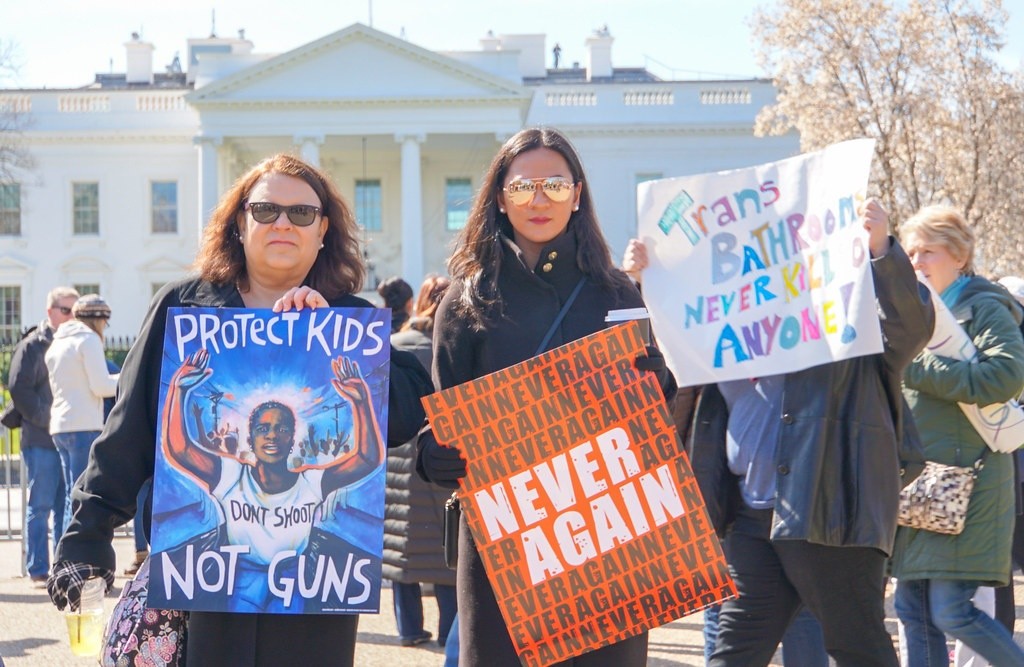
[1,399,22,429]
[897,444,991,534]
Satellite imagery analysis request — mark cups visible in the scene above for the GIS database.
[64,574,107,659]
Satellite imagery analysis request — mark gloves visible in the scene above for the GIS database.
[636,346,666,390]
[47,563,114,612]
[421,439,468,489]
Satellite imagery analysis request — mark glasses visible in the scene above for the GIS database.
[504,177,576,205]
[52,305,73,314]
[241,202,323,227]
[103,317,110,329]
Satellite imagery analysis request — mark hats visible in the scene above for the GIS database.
[71,293,111,318]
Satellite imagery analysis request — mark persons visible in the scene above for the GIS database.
[993,277,1023,637]
[44,152,436,667]
[7,286,83,592]
[43,294,123,549]
[883,205,1024,667]
[415,126,680,667]
[620,199,935,667]
[375,275,413,335]
[385,276,460,649]
